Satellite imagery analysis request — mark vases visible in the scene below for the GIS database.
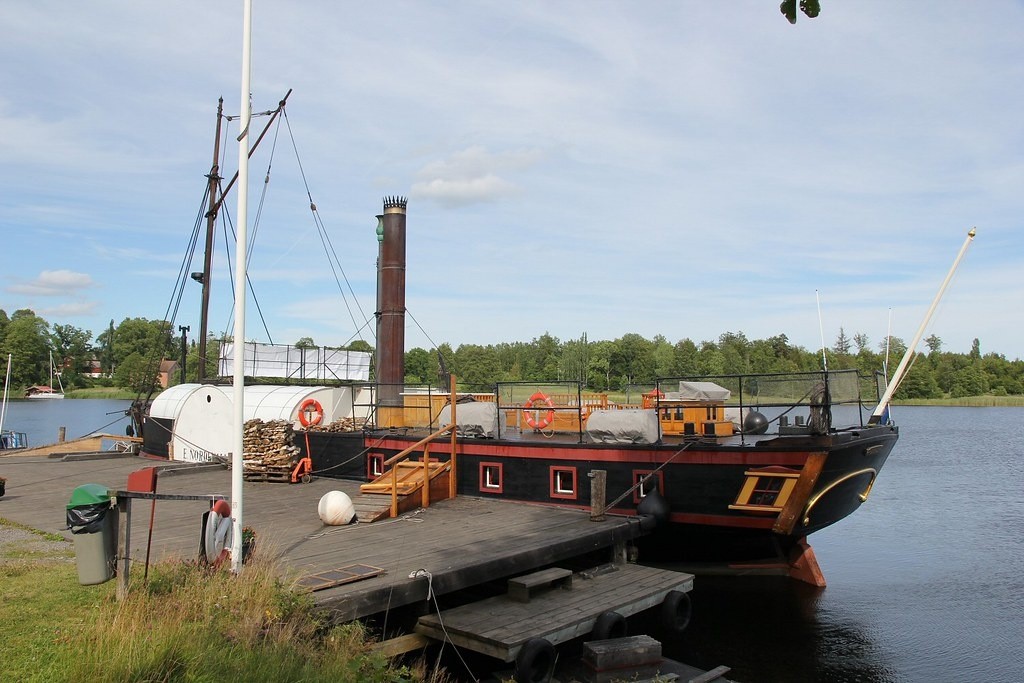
[242,543,255,562]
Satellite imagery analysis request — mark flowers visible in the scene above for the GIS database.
[241,527,257,547]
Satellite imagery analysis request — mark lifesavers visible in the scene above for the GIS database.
[299,399,322,426]
[515,639,557,683]
[524,393,555,429]
[665,591,691,631]
[594,612,627,640]
[206,500,231,566]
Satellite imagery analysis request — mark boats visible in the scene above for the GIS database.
[93,84,978,614]
[1,353,144,456]
[25,350,65,400]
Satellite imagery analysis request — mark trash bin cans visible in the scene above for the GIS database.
[65,483,117,585]
